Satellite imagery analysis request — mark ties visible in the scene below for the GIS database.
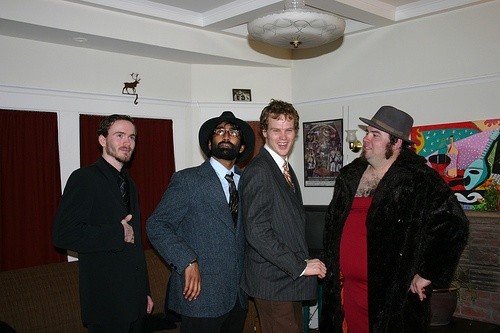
[118,173,131,213]
[282,161,295,193]
[225,172,239,227]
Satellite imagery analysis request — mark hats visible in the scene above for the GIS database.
[359,106,415,144]
[199,110,256,162]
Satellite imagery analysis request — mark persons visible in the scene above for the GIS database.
[237,99,328,333]
[317,105,470,333]
[146,111,251,333]
[52,114,154,333]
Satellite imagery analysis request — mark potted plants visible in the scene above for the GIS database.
[431,268,481,326]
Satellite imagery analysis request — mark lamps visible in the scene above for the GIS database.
[247,0,346,49]
[344,129,362,153]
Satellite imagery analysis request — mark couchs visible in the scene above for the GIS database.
[0,248,181,333]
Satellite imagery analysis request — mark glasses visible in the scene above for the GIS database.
[214,128,242,137]
